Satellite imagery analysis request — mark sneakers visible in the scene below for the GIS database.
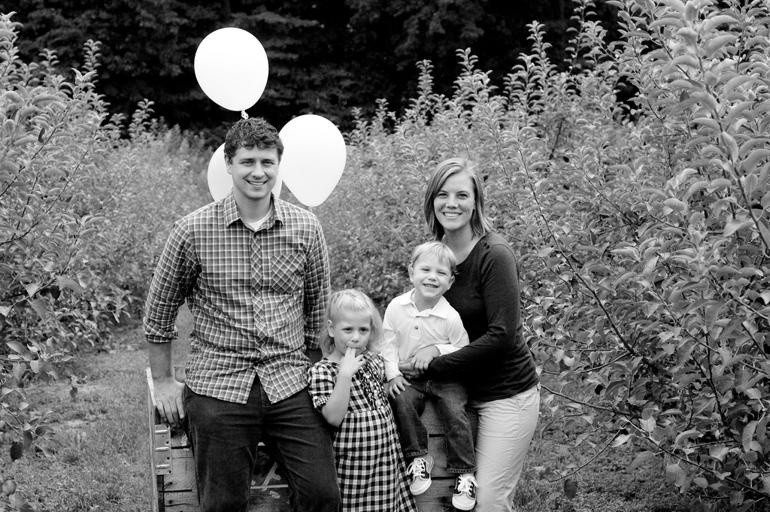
[406,455,434,496]
[451,473,479,511]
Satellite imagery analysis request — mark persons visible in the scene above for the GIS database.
[423,157,541,512]
[308,289,420,512]
[142,117,342,512]
[382,241,478,511]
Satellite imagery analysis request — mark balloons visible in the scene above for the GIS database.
[193,27,270,120]
[277,114,346,207]
[207,142,282,203]
